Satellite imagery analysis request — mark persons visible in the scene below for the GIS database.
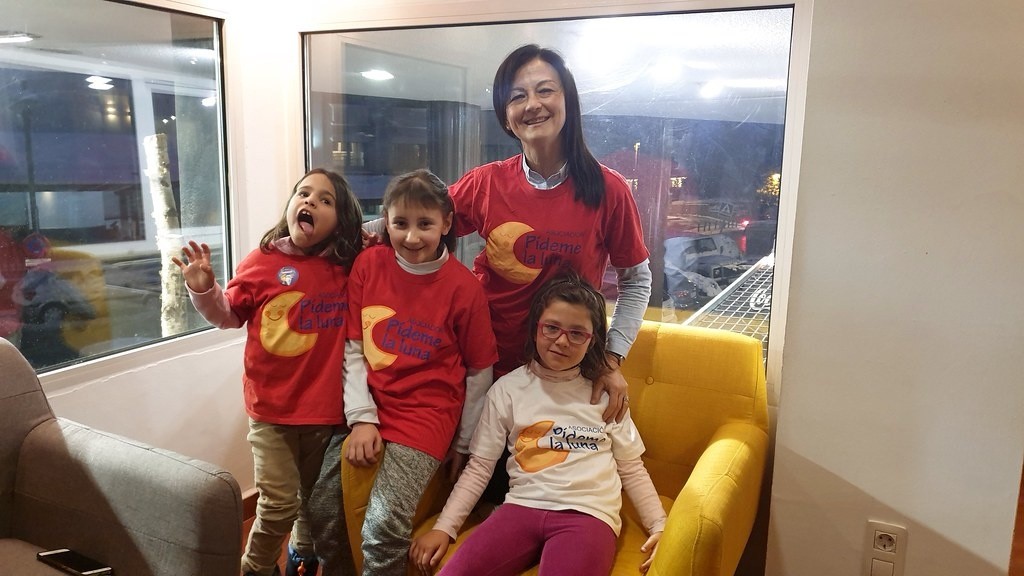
[171,43,667,576]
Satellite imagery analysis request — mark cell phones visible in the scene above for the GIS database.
[37,548,112,576]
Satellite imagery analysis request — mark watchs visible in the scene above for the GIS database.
[605,351,625,366]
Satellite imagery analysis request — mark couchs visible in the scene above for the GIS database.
[0,337,244,576]
[344,320,772,576]
[49,250,113,357]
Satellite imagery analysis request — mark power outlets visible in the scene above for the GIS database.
[861,520,909,576]
